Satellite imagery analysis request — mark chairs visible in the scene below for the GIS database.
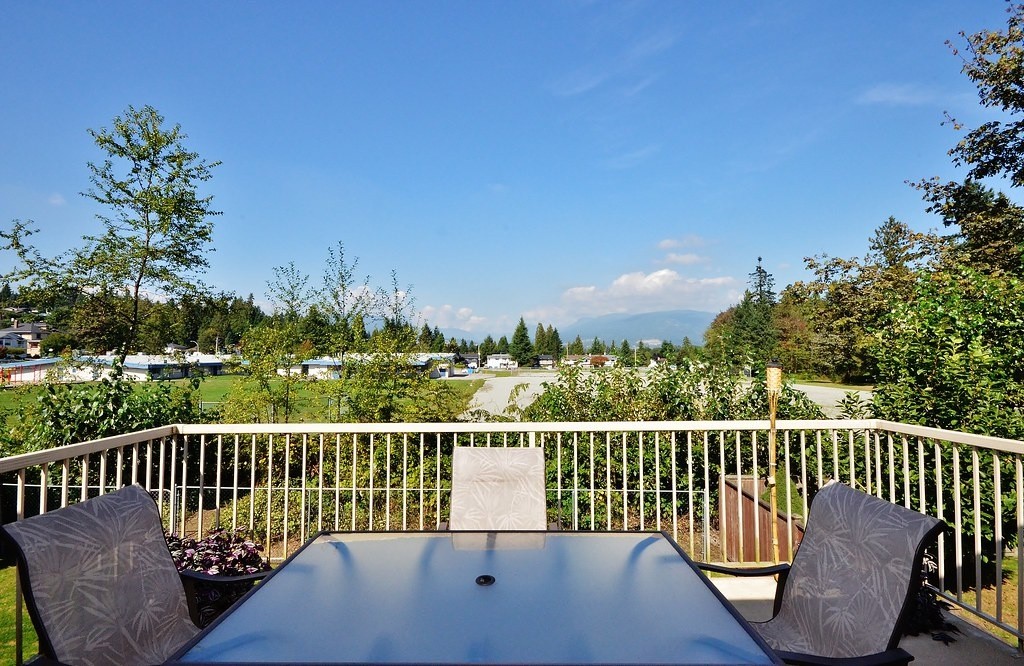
[3,482,203,666]
[693,479,947,666]
[438,445,563,532]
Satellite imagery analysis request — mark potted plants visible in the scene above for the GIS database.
[168,528,274,630]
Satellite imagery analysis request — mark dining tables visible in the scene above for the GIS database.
[161,527,789,666]
